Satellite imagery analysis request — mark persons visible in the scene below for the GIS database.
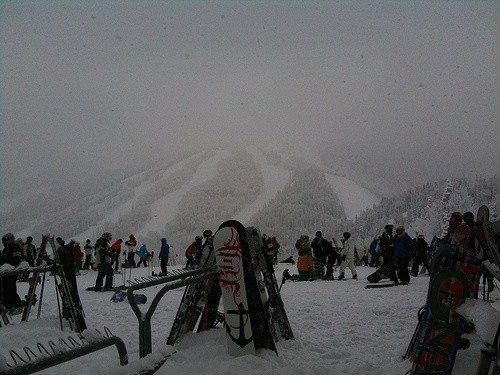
[184,211,500,292]
[0,233,178,316]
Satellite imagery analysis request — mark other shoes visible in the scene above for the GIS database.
[322,274,335,281]
[105,286,115,290]
[402,281,408,284]
[95,287,103,292]
[400,281,404,284]
[161,273,167,276]
[352,274,357,279]
[338,274,344,279]
[3,307,15,312]
[12,306,23,315]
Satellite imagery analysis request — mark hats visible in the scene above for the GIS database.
[396,225,405,235]
[385,225,395,230]
[343,232,350,236]
[417,232,426,238]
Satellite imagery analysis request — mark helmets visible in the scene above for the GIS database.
[2,233,14,247]
[203,230,212,237]
[101,232,112,242]
[195,236,203,240]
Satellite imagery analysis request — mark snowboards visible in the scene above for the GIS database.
[366,259,397,283]
[405,204,500,375]
[212,220,295,359]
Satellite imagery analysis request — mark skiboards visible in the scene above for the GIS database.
[85,286,119,292]
[166,234,221,346]
[0,233,86,333]
[365,283,408,289]
